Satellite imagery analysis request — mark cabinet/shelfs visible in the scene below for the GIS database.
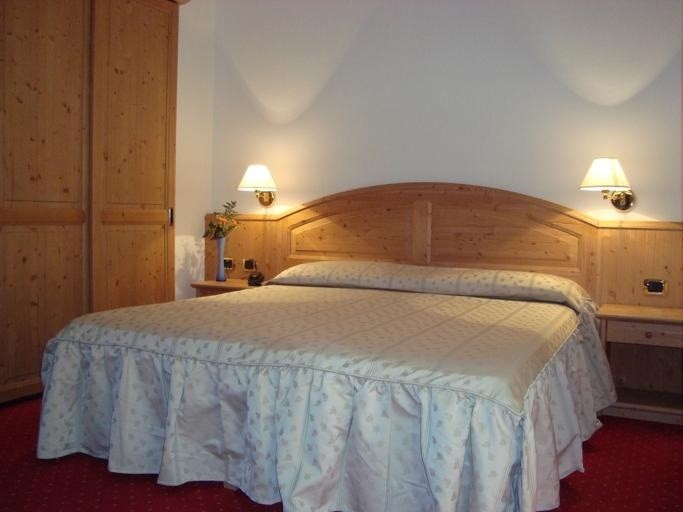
[0,0,179,403]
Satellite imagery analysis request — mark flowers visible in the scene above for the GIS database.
[202,200,249,240]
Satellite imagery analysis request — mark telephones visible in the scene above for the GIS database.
[248,272,264,285]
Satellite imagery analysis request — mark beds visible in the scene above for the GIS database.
[35,182,599,511]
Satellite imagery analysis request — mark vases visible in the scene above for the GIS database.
[216,238,226,281]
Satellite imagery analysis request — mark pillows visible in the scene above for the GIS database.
[261,260,599,334]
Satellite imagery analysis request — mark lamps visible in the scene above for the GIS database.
[577,157,635,212]
[237,165,277,207]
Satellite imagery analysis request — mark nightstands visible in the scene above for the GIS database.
[595,304,683,425]
[190,279,263,297]
